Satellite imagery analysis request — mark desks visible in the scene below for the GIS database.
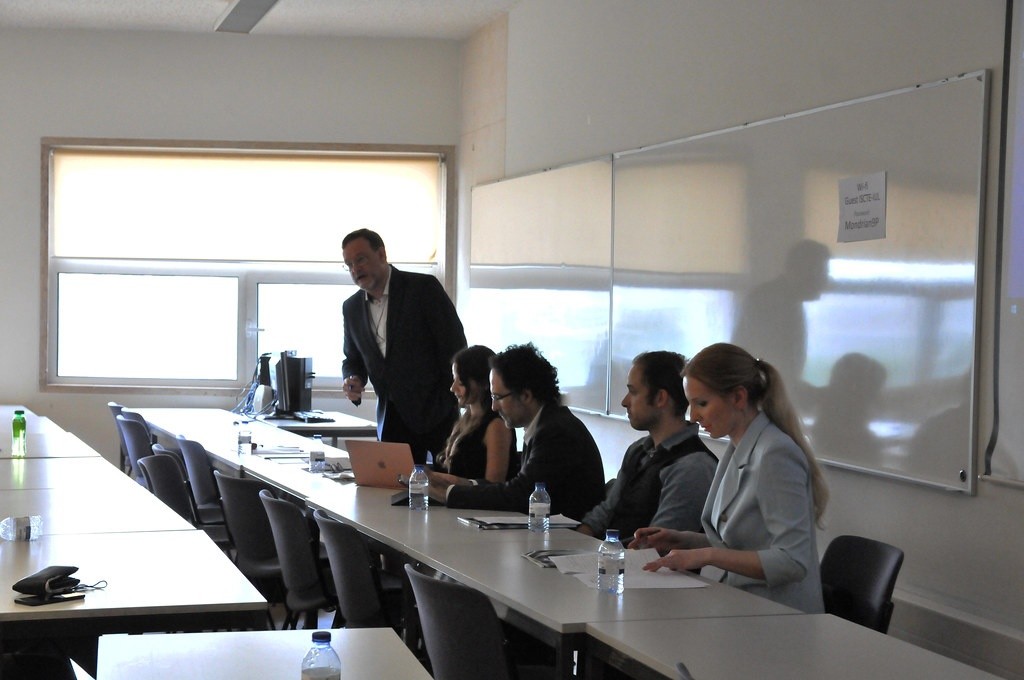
[0,406,1024,680]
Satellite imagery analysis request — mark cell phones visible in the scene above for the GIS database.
[14,592,86,607]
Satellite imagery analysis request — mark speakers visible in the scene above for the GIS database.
[253,385,273,415]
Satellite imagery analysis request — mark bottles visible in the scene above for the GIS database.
[309,435,325,475]
[408,464,429,510]
[0,515,43,542]
[598,529,625,594]
[529,483,551,534]
[12,410,26,456]
[301,632,342,680]
[238,421,252,458]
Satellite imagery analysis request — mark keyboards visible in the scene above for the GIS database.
[293,411,335,423]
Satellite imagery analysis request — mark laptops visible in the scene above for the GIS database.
[345,440,416,490]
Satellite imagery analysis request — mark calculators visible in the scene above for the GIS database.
[292,411,338,422]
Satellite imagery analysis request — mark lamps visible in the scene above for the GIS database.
[213,0,279,34]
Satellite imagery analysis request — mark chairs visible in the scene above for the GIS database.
[109,402,156,478]
[174,434,236,542]
[312,509,424,655]
[820,532,904,627]
[119,408,152,441]
[259,488,344,630]
[134,455,237,551]
[211,468,282,629]
[116,414,195,513]
[402,564,511,680]
[150,443,197,520]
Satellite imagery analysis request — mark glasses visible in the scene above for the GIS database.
[343,253,374,272]
[490,392,514,401]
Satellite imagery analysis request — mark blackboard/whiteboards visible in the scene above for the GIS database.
[607,67,989,497]
[468,153,613,418]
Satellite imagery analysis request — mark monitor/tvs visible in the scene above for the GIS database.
[264,352,296,420]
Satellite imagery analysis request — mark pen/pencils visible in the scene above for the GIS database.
[349,375,353,390]
[620,529,661,545]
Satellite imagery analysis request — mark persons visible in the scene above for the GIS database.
[342,228,467,465]
[432,345,517,482]
[575,351,719,575]
[415,342,606,541]
[628,343,830,615]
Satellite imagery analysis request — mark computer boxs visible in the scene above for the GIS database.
[259,353,313,416]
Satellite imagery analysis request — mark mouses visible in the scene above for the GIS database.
[312,410,323,414]
